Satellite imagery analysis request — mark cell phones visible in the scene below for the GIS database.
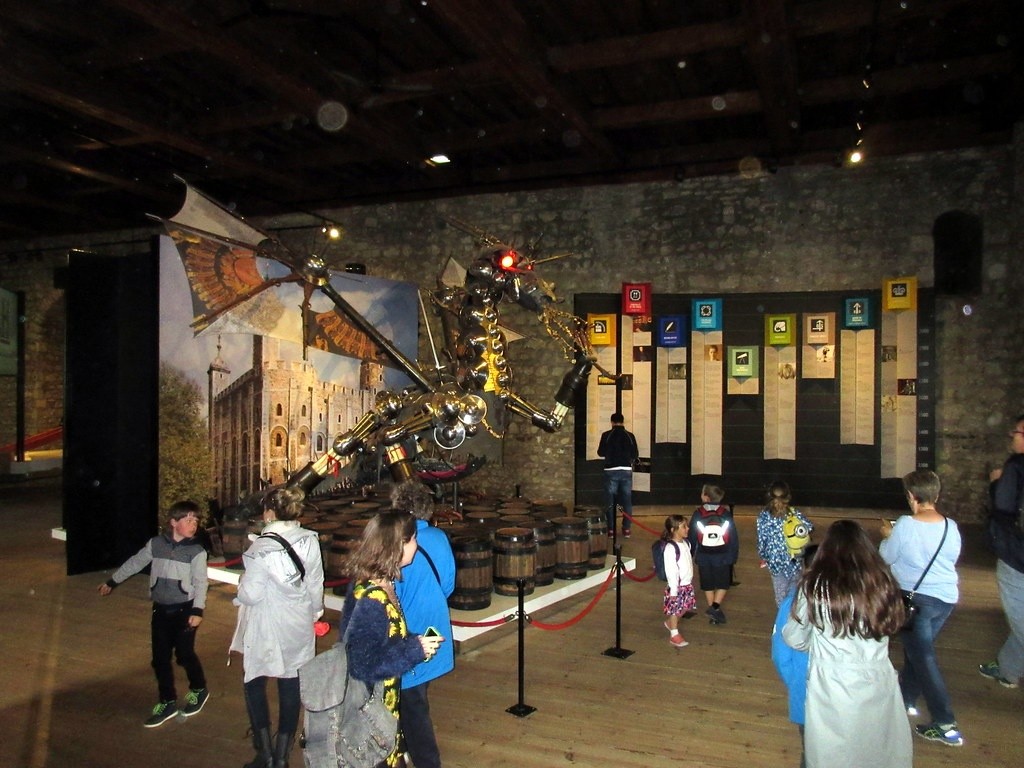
[889,520,896,527]
[423,626,442,662]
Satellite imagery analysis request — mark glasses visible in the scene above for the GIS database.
[1011,429,1024,437]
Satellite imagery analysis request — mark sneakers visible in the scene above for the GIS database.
[181,688,209,717]
[915,721,962,746]
[978,657,1018,688]
[664,620,672,632]
[669,635,689,647]
[904,702,916,717]
[144,698,178,729]
[704,607,726,625]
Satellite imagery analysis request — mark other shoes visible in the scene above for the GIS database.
[608,529,617,538]
[622,527,631,538]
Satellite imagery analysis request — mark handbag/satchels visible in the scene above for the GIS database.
[899,594,915,631]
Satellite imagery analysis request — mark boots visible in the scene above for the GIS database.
[271,730,295,768]
[243,725,275,768]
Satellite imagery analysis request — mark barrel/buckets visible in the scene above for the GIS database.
[492,527,537,597]
[448,536,494,611]
[574,511,608,570]
[547,516,590,581]
[297,495,391,597]
[222,513,250,569]
[245,518,265,550]
[438,498,566,536]
[517,522,558,586]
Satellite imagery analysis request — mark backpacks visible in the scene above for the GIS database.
[652,538,689,580]
[696,505,729,554]
[297,585,397,768]
[783,507,811,555]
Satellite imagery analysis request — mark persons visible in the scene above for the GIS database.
[878,470,962,747]
[757,480,812,604]
[772,546,822,767]
[686,485,740,625]
[339,508,445,768]
[977,415,1024,690]
[227,487,325,768]
[98,501,208,729]
[390,481,455,768]
[661,515,695,647]
[783,520,914,768]
[598,413,638,539]
